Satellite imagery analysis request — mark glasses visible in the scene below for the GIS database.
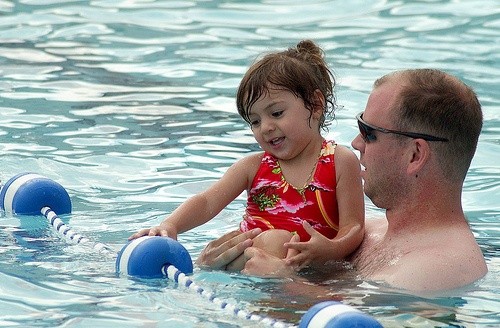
[355,111,449,144]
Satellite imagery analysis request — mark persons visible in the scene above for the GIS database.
[127,41,365,272]
[195,70,488,299]
